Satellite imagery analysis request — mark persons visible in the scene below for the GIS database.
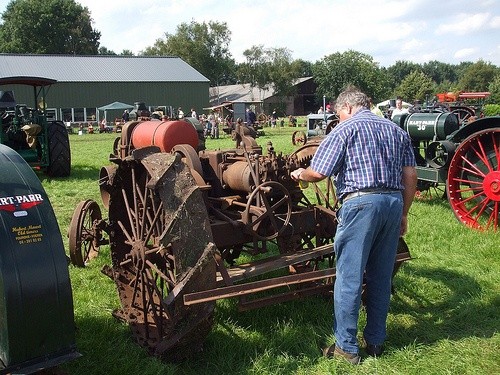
[89,107,279,138]
[318,99,410,121]
[290,90,417,365]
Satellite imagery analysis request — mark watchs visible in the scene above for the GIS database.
[297,169,304,180]
